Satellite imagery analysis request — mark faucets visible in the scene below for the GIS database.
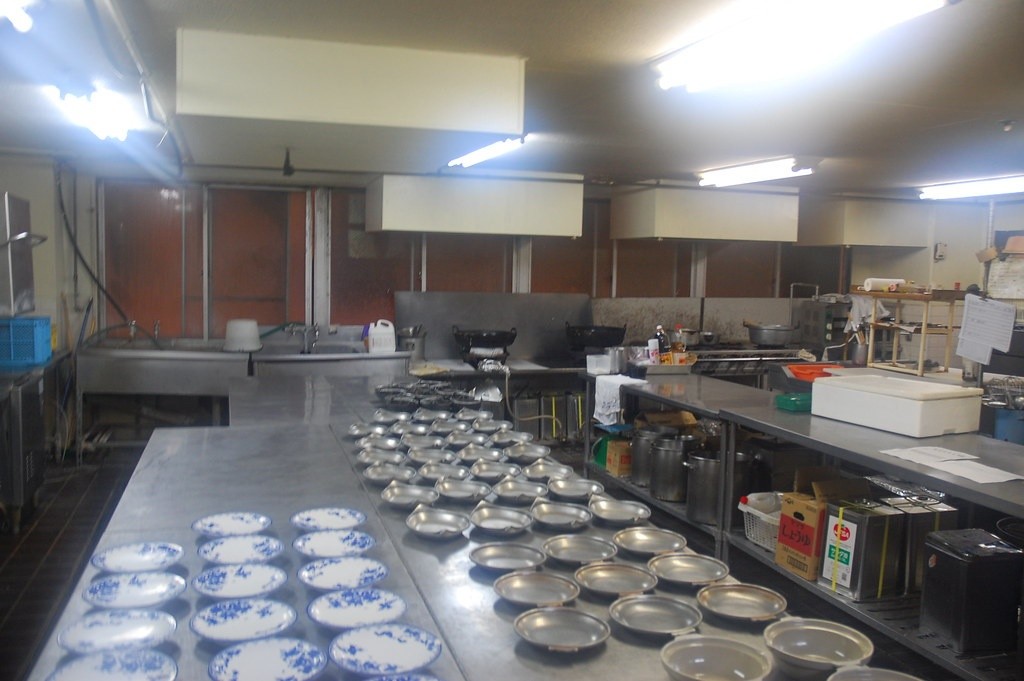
[282,321,321,352]
[126,318,138,341]
[152,319,161,341]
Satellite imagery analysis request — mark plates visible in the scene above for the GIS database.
[347,406,787,653]
[43,507,442,681]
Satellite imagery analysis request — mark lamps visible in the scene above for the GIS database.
[447,130,529,169]
[917,175,1024,202]
[697,155,817,187]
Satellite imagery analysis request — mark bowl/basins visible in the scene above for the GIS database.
[700,332,721,346]
[375,380,482,412]
[586,354,610,375]
[397,325,417,338]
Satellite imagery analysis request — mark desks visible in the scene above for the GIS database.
[33,376,1024,680]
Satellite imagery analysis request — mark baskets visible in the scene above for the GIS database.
[0,315,51,366]
[738,492,786,553]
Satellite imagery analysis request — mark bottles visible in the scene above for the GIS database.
[605,325,686,374]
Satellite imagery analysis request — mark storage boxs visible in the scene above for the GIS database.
[635,406,697,431]
[776,463,872,582]
[0,316,52,368]
[810,374,985,437]
[606,439,632,478]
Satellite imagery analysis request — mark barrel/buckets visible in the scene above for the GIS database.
[648,435,704,502]
[222,318,264,353]
[629,430,678,488]
[368,320,395,353]
[682,449,756,526]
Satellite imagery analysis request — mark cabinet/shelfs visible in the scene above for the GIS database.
[869,293,965,377]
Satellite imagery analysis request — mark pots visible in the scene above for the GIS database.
[666,328,699,346]
[742,320,800,345]
[452,325,517,347]
[565,321,627,347]
[659,616,922,681]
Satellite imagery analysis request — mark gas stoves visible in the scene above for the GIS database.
[674,337,810,373]
[460,347,510,370]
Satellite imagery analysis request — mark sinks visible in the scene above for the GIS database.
[262,342,362,357]
[101,338,224,353]
